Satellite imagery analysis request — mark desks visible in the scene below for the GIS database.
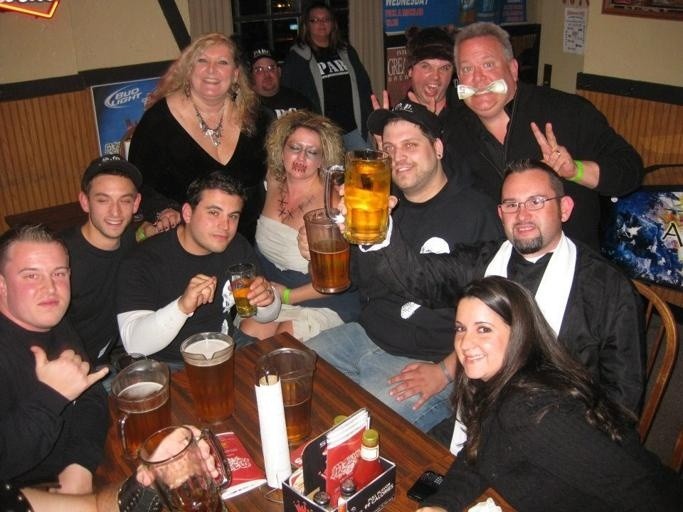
[18,330,522,512]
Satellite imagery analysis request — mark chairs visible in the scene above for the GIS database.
[627,275,677,446]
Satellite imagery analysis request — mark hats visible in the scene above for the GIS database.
[366,99,446,139]
[248,46,279,72]
[401,26,455,74]
[80,153,145,198]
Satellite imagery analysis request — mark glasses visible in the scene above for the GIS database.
[251,64,278,74]
[498,195,566,214]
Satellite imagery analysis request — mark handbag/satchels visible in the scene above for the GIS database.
[593,160,682,296]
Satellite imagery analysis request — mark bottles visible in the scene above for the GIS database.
[458,0,497,25]
[333,415,347,425]
[339,430,385,506]
[314,492,330,507]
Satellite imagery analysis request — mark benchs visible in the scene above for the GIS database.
[3,191,148,242]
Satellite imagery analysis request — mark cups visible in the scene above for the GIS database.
[138,425,237,512]
[110,359,172,462]
[302,208,354,294]
[324,149,389,243]
[256,344,316,448]
[228,261,258,319]
[181,329,236,424]
